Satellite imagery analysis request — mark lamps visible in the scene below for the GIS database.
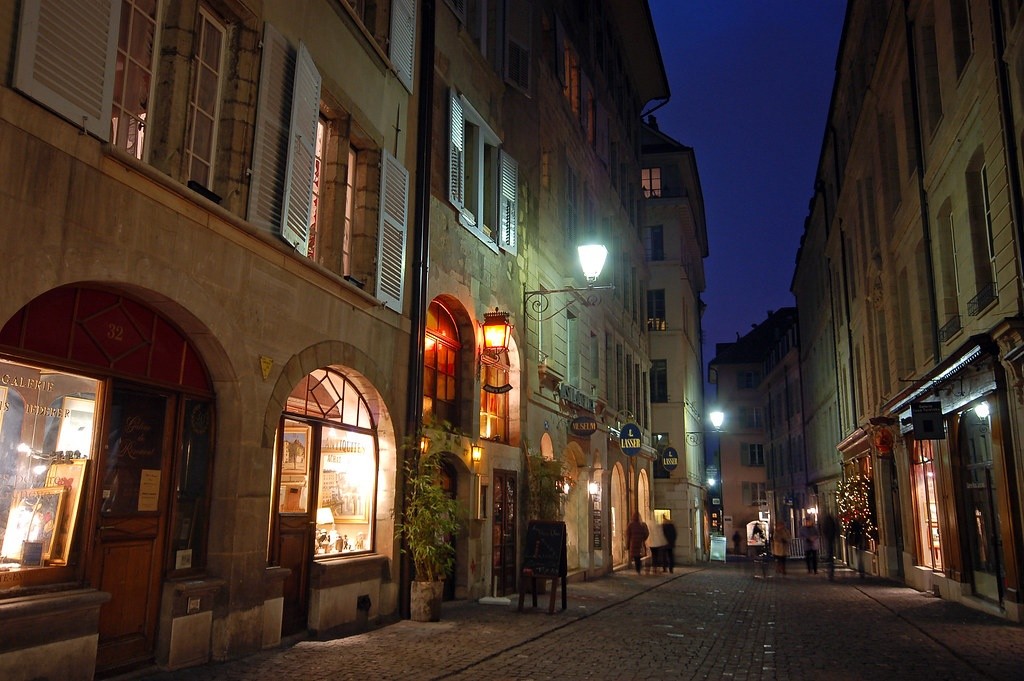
[686,411,727,447]
[476,307,515,381]
[523,242,615,333]
[974,389,998,423]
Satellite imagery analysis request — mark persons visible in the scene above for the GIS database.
[752,522,765,539]
[799,516,820,576]
[771,516,792,575]
[645,512,677,574]
[314,528,364,555]
[625,512,648,575]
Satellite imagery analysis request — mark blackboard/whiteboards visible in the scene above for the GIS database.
[519,521,565,579]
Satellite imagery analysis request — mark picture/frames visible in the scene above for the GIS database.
[279,426,309,476]
[44,458,91,564]
[318,447,370,524]
[1,486,67,560]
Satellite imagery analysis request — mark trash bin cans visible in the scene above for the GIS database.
[710,534,727,564]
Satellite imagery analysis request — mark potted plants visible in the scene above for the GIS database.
[390,417,468,623]
[519,440,578,594]
